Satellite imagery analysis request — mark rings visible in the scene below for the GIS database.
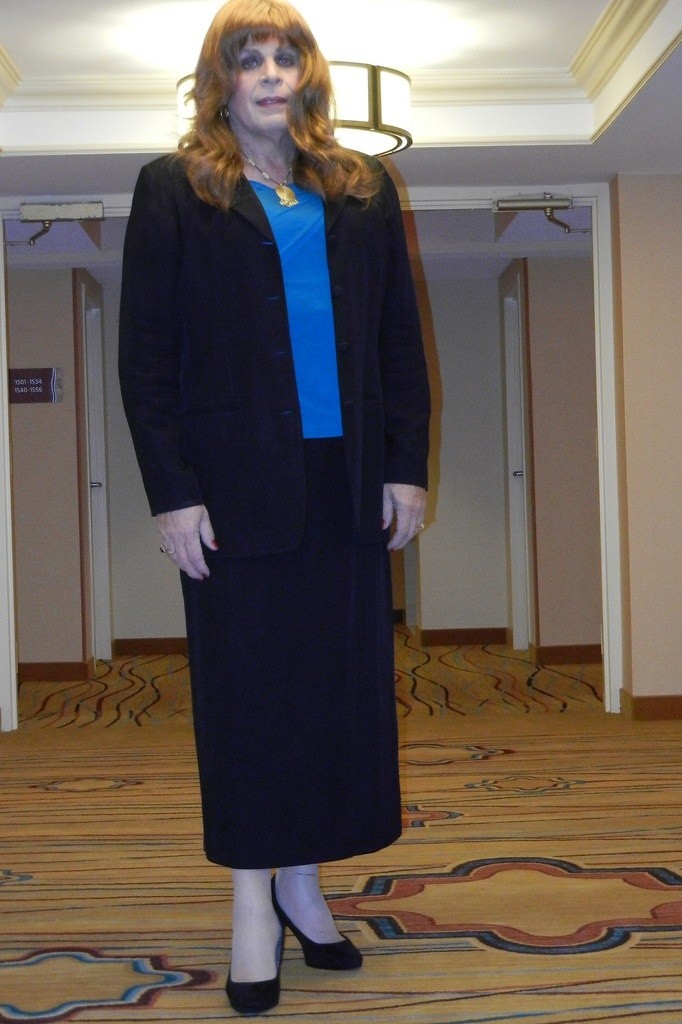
[418,523,424,530]
[159,543,175,554]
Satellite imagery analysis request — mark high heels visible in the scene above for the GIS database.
[227,924,285,1014]
[267,874,363,970]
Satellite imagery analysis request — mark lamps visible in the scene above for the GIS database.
[319,61,415,158]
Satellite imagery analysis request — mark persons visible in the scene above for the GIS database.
[118,1,431,1013]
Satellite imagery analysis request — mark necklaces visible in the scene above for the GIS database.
[240,148,299,208]
